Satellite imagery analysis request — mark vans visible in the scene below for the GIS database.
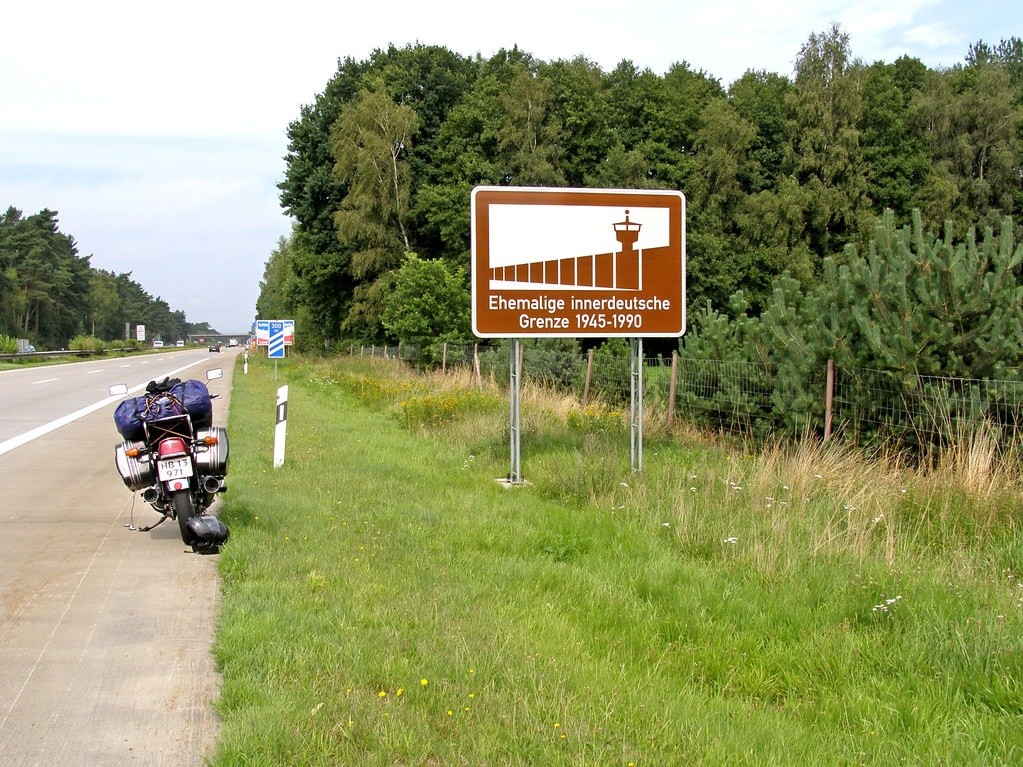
[176,340,185,347]
[154,341,163,348]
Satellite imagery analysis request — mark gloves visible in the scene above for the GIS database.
[145,376,172,395]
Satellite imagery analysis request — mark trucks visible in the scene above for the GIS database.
[229,339,236,347]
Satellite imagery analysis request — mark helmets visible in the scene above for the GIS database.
[184,513,228,555]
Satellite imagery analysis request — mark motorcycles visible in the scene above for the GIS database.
[110,369,229,543]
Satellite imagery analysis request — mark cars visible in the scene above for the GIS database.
[208,343,220,352]
